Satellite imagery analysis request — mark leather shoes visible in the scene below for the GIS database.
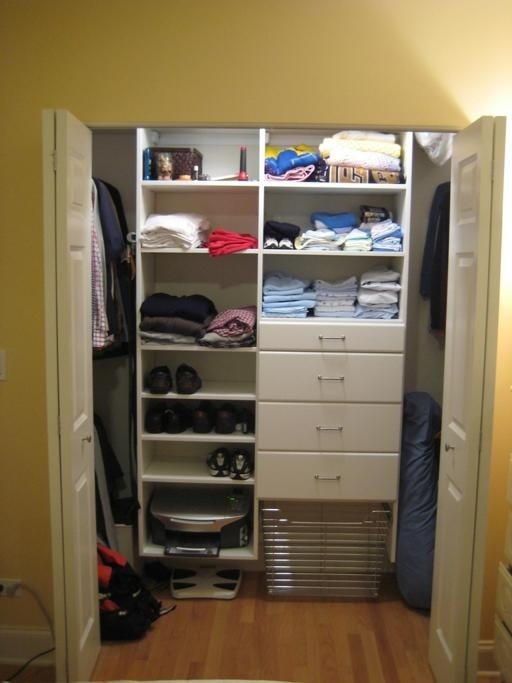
[145,398,241,436]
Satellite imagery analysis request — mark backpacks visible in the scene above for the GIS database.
[96,542,166,642]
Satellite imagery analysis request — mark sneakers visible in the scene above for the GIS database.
[226,448,254,480]
[173,363,202,395]
[205,446,232,479]
[264,236,294,249]
[149,364,172,395]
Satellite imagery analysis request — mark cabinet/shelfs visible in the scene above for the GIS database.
[130,123,419,574]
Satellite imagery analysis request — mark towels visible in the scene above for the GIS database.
[140,211,210,250]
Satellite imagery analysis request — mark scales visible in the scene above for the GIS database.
[170,559,243,600]
